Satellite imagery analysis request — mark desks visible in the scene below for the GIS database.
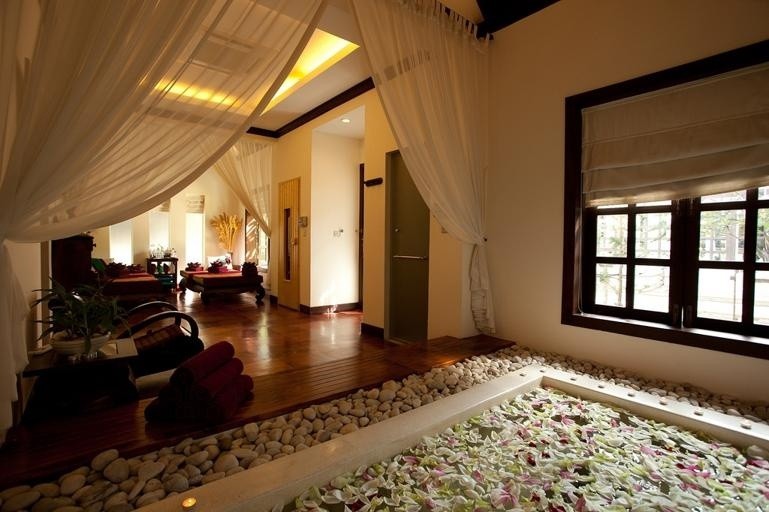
[99,265,267,306]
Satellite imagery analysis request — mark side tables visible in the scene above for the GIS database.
[19,337,140,407]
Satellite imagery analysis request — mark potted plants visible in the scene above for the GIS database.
[20,270,132,356]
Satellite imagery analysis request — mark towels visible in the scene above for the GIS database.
[143,340,255,432]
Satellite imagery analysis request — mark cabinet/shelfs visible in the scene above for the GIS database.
[146,257,178,291]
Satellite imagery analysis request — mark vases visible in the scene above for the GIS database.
[224,251,234,270]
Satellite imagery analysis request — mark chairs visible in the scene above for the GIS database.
[113,300,204,377]
